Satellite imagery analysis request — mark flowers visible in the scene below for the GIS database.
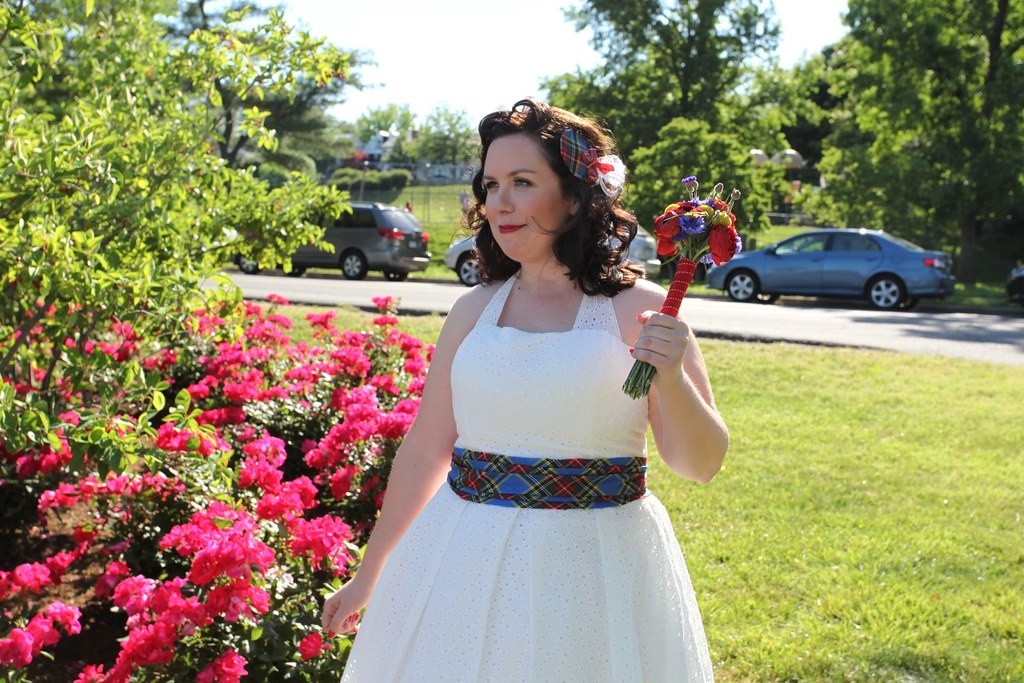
[611,177,741,400]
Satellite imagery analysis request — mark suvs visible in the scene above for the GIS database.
[232,201,432,283]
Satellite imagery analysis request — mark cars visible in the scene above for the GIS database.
[706,229,957,312]
[426,174,449,183]
[442,217,663,281]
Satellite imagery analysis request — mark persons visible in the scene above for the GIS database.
[461,187,474,223]
[406,202,412,212]
[1007,260,1024,303]
[322,99,729,683]
